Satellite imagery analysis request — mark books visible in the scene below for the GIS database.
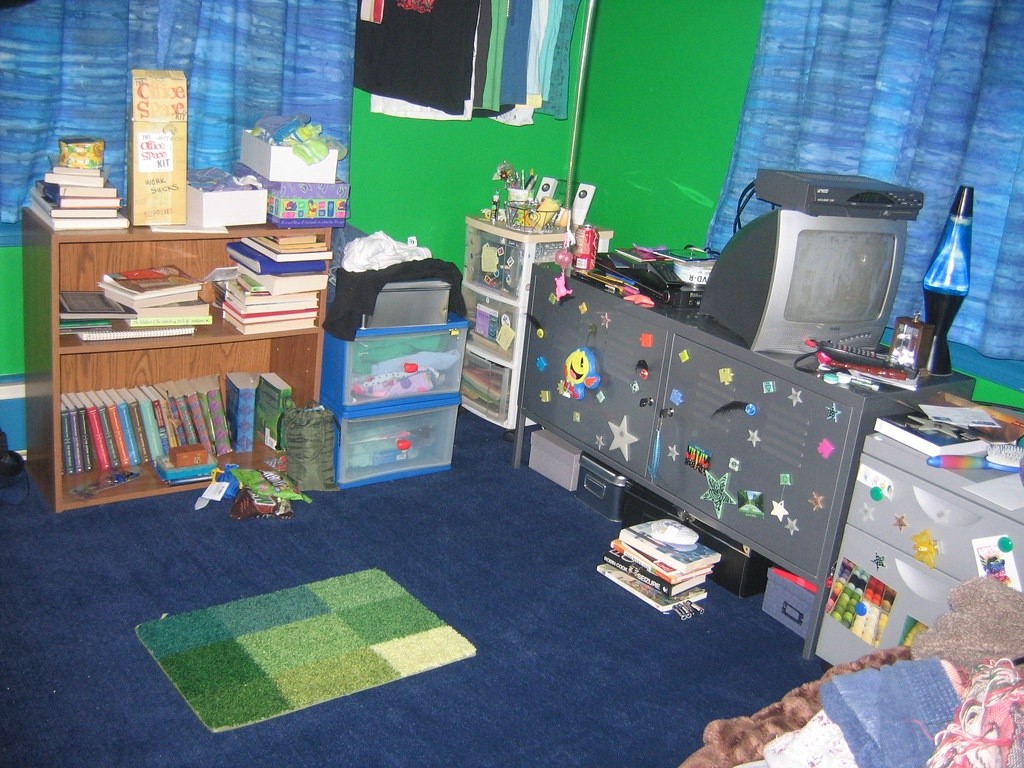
[595,521,721,612]
[59,235,331,342]
[29,155,130,232]
[874,410,1010,458]
[606,247,709,268]
[60,372,291,487]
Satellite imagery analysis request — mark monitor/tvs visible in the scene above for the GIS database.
[699,209,906,357]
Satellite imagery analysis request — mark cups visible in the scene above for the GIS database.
[507,187,528,204]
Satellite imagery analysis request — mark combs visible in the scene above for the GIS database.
[926,444,1024,472]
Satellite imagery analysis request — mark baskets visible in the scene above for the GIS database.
[504,201,561,233]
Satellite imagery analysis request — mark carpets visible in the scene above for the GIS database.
[132,566,479,732]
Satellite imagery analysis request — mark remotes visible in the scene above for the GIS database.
[820,341,888,367]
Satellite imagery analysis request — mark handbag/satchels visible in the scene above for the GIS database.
[284,396,340,492]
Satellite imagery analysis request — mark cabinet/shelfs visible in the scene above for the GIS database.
[459,215,615,430]
[511,260,977,660]
[815,432,1024,667]
[21,208,332,515]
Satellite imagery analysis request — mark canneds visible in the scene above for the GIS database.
[571,225,600,270]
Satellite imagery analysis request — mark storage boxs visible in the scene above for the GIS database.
[317,399,463,489]
[320,314,471,411]
[241,130,340,184]
[233,161,352,228]
[365,281,451,329]
[760,567,816,640]
[189,187,269,228]
[618,477,771,598]
[575,451,625,524]
[528,432,581,491]
[130,64,188,226]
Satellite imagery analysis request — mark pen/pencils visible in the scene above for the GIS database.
[515,169,538,190]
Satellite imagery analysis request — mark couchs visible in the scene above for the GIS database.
[643,579,1024,768]
